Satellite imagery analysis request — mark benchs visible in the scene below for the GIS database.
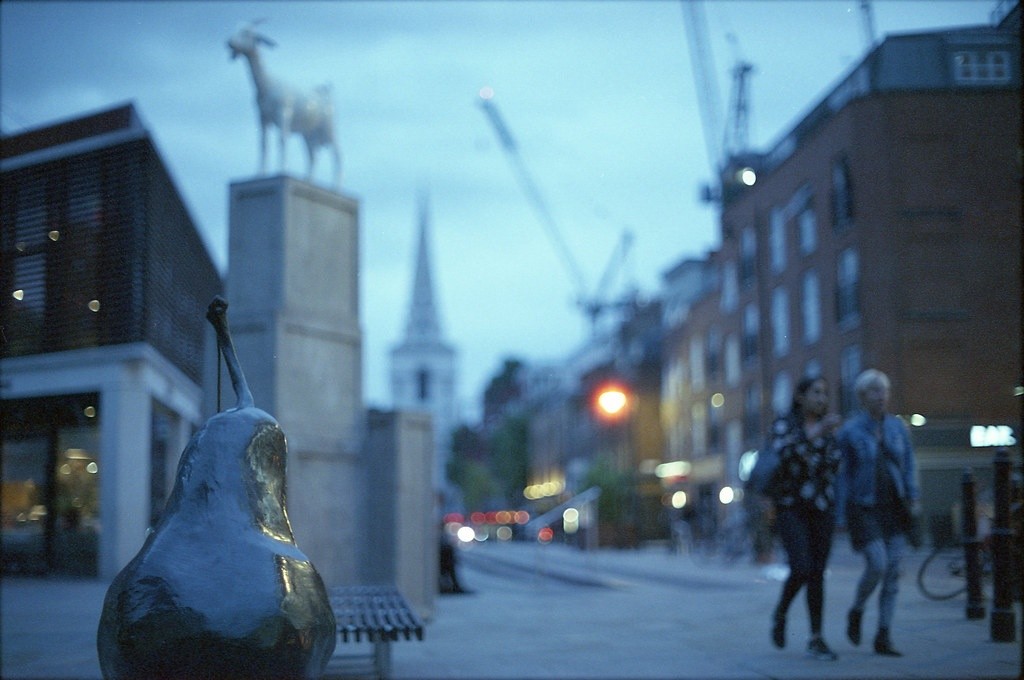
[326,583,425,680]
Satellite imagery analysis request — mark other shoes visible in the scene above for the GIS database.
[805,637,837,661]
[874,627,901,656]
[847,608,861,645]
[772,617,785,647]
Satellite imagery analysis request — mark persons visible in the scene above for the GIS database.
[437,487,477,595]
[761,376,842,661]
[832,369,920,657]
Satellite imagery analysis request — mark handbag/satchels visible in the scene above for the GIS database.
[894,495,927,547]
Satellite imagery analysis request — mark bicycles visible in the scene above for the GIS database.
[918,534,999,601]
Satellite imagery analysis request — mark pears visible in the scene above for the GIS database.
[97,293,336,680]
